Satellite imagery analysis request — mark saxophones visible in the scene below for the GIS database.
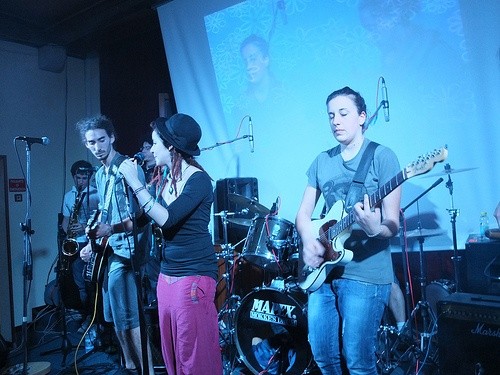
[61,185,82,256]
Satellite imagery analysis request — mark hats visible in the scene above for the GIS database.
[155,114,202,156]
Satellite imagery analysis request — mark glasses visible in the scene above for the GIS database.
[138,146,152,151]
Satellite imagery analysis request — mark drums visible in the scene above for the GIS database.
[230,279,317,375]
[241,215,299,273]
[213,243,234,274]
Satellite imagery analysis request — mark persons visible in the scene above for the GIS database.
[140,140,160,304]
[119,113,223,375]
[64,160,99,333]
[79,117,154,375]
[241,36,277,104]
[296,88,405,375]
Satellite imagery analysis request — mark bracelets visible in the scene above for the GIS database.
[134,185,146,194]
[140,197,156,213]
[110,224,113,235]
[367,227,381,237]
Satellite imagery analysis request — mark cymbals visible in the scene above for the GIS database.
[395,229,446,238]
[227,217,251,227]
[226,192,270,215]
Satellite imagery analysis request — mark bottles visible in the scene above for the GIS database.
[479,212,491,242]
[84,328,95,353]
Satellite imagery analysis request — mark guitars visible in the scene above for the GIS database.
[294,146,448,286]
[81,209,109,283]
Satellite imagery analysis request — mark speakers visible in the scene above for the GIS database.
[435,292,500,375]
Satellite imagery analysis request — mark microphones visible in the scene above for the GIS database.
[114,152,145,185]
[79,166,99,172]
[382,78,390,122]
[249,117,255,152]
[17,136,49,145]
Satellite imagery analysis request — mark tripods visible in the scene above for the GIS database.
[211,212,250,375]
[372,177,443,375]
[39,227,87,367]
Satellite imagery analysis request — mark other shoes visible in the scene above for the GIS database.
[397,333,420,350]
[113,366,140,375]
